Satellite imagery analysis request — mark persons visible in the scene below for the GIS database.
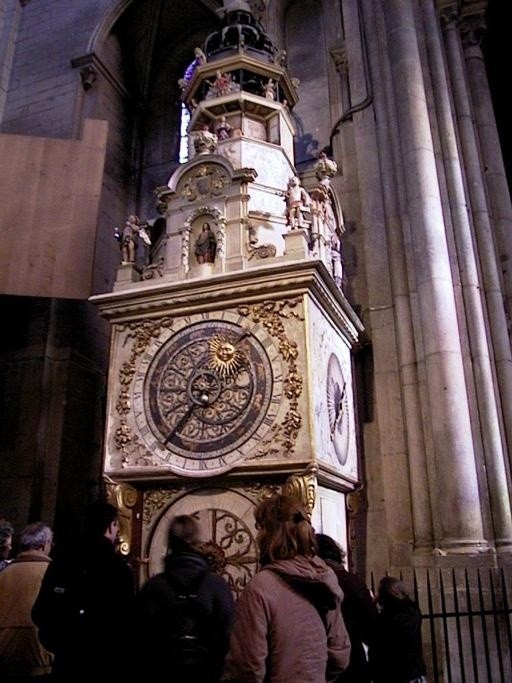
[114,44,347,291]
[32,502,142,682]
[315,532,425,683]
[0,519,51,683]
[134,514,238,683]
[220,498,350,682]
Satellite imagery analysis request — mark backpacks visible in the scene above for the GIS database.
[169,615,209,665]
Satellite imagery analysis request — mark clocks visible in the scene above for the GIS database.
[117,305,304,480]
